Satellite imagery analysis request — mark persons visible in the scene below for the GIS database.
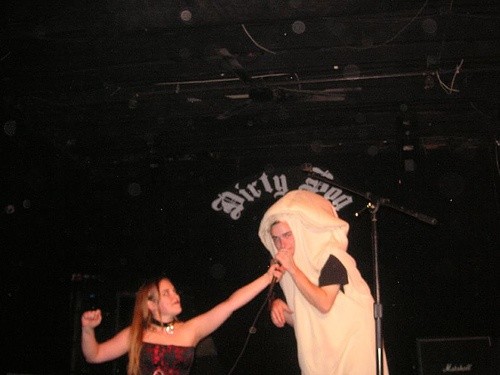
[257,190,389,375]
[81,264,284,374]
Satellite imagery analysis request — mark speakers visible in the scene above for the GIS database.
[414,336,495,375]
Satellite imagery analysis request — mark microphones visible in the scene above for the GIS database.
[272,260,281,289]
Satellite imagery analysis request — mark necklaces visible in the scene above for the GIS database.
[151,318,176,335]
